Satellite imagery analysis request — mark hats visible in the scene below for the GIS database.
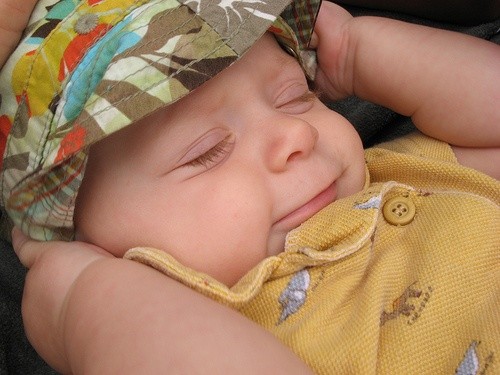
[1,0,321,242]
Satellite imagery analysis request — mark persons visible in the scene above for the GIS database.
[1,0,500,374]
[1,0,422,375]
